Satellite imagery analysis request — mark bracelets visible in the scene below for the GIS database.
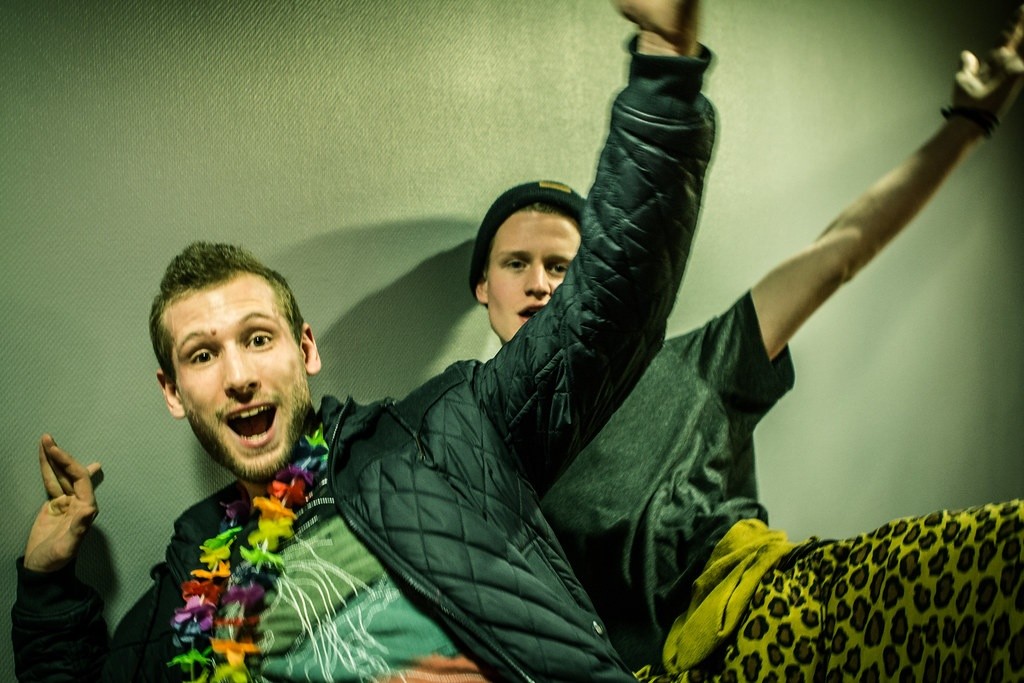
[940,104,1001,139]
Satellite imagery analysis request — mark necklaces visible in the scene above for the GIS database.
[164,419,328,683]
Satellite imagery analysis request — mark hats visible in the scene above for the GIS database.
[469,180,592,307]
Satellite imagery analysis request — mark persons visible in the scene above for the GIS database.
[11,0,713,683]
[471,1,1024,683]
